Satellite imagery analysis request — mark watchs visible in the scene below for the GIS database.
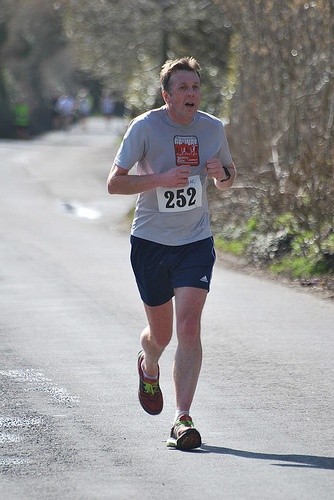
[220,166,231,182]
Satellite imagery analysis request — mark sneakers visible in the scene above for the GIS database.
[137,350,163,415]
[167,414,202,450]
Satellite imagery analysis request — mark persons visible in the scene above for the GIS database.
[106,56,236,450]
